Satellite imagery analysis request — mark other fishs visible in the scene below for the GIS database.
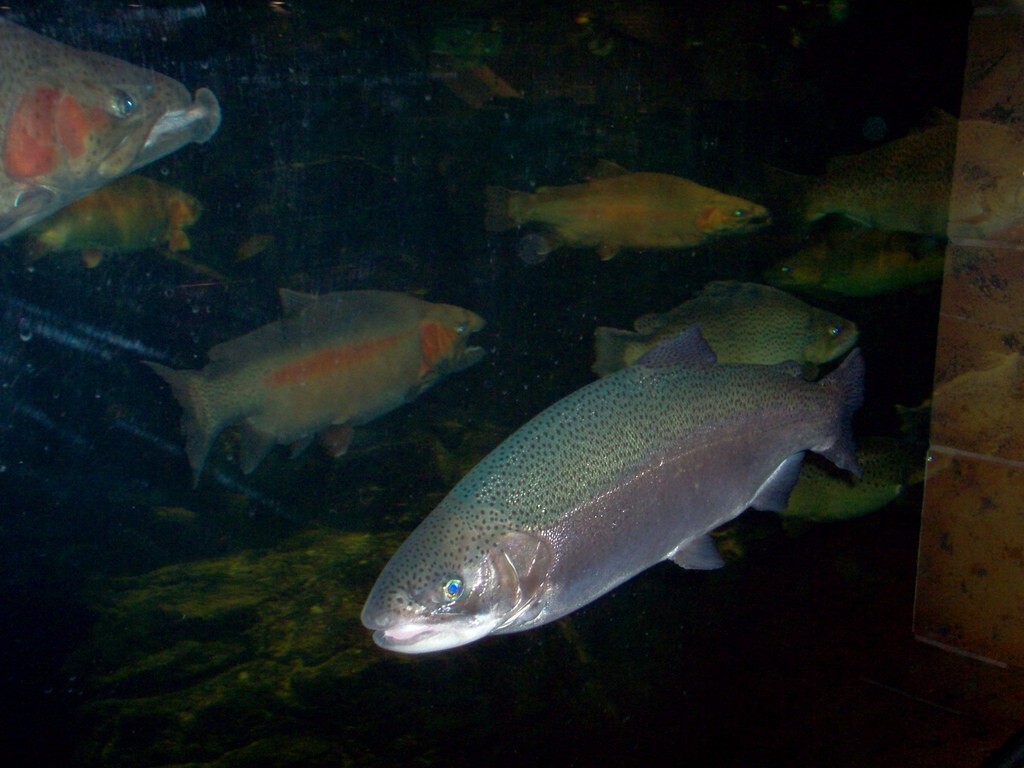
[0,21,877,654]
